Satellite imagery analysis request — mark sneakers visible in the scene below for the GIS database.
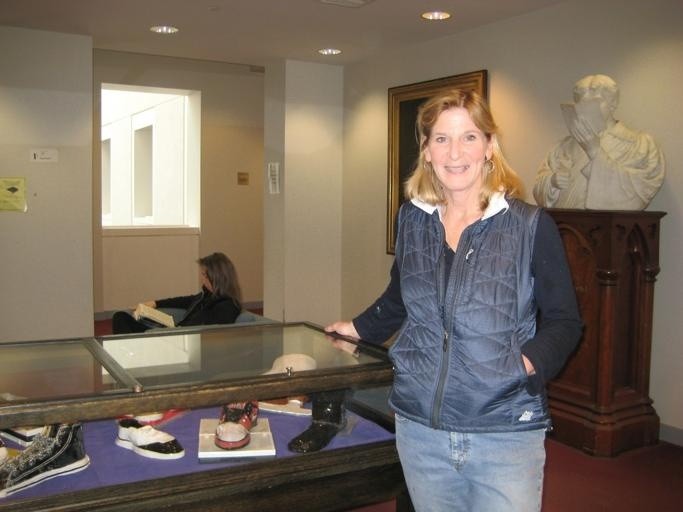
[215,401,258,450]
[0,423,91,498]
[115,418,185,460]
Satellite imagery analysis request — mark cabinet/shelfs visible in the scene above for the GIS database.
[537,207,667,448]
[0,321,414,512]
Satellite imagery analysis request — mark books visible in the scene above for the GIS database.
[561,92,607,135]
[133,302,175,329]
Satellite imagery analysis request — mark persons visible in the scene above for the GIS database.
[528,76,667,209]
[112,252,242,335]
[323,87,587,512]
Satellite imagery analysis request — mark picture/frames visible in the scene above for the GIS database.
[387,70,488,255]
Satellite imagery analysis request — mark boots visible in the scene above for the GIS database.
[288,390,347,453]
